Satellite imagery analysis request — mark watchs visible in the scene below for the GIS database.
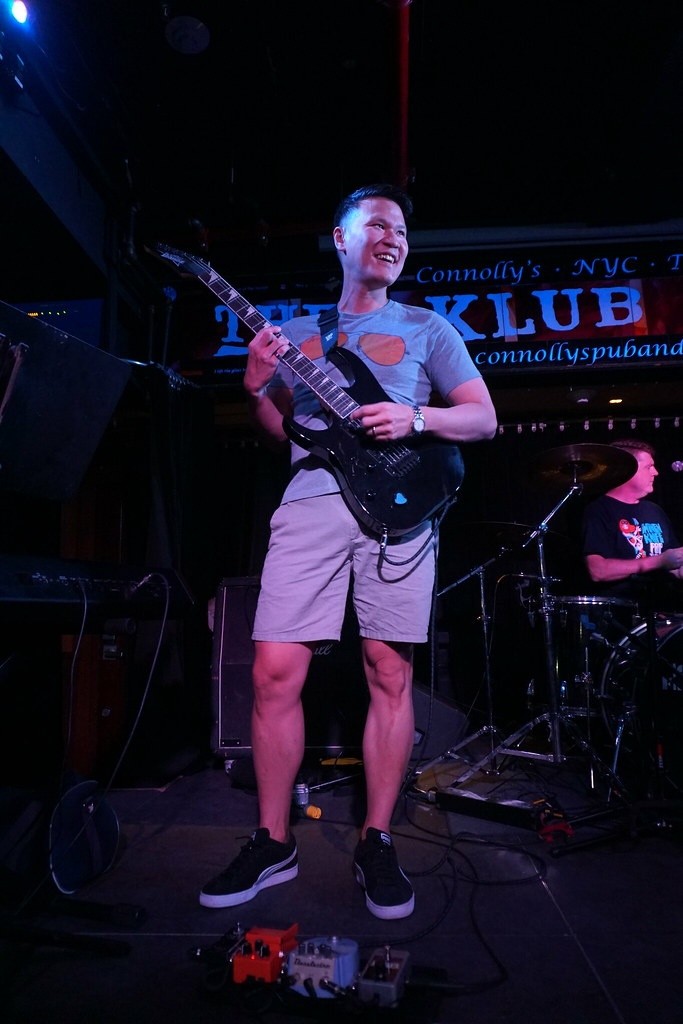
[410,405,426,438]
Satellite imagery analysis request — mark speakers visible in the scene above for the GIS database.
[211,577,372,761]
[408,680,484,761]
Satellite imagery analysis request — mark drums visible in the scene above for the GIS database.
[596,615,682,788]
[537,591,641,694]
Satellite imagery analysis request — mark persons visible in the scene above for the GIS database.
[583,441,682,613]
[198,187,499,924]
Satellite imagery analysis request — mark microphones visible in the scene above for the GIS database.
[509,574,563,583]
[671,461,683,472]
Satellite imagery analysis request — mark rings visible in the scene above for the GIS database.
[274,352,281,359]
[372,427,376,436]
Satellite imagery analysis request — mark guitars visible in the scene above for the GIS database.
[155,242,464,540]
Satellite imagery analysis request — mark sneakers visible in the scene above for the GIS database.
[353,826,416,919]
[198,827,298,908]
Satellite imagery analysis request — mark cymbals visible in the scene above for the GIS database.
[532,442,639,498]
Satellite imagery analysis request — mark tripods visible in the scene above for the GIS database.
[408,467,639,812]
